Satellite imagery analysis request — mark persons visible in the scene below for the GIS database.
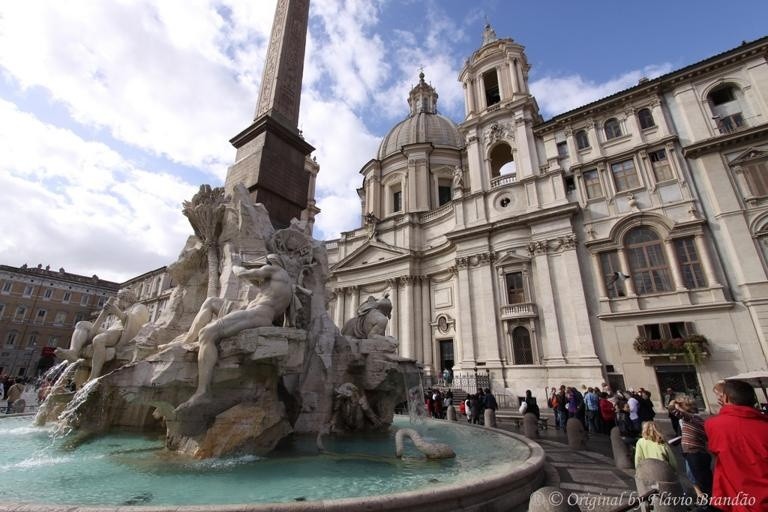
[52,288,151,388]
[451,164,465,186]
[341,293,400,347]
[393,364,725,512]
[0,373,76,415]
[703,381,768,511]
[156,253,295,414]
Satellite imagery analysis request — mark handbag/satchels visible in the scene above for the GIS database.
[518,402,527,415]
